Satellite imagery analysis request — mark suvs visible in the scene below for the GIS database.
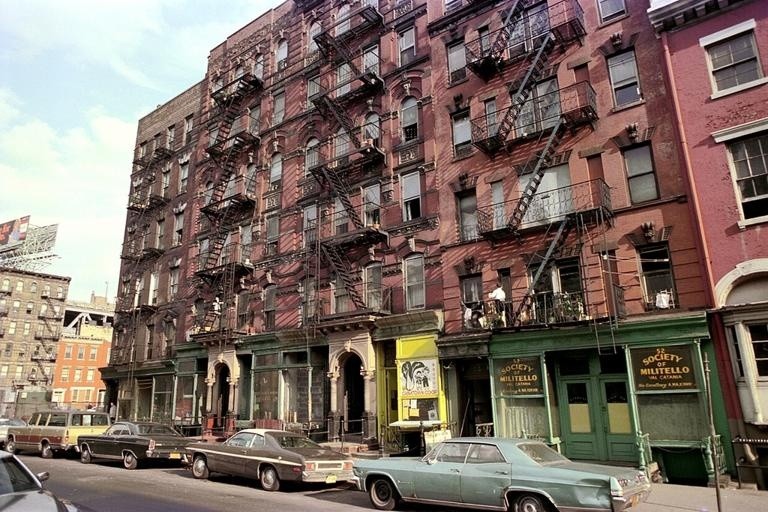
[7,411,111,458]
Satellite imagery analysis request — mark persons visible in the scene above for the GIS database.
[108,401,117,424]
[488,280,506,314]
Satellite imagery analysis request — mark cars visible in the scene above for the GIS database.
[353,434,651,511]
[0,449,98,512]
[0,415,29,448]
[77,422,190,469]
[185,427,354,491]
